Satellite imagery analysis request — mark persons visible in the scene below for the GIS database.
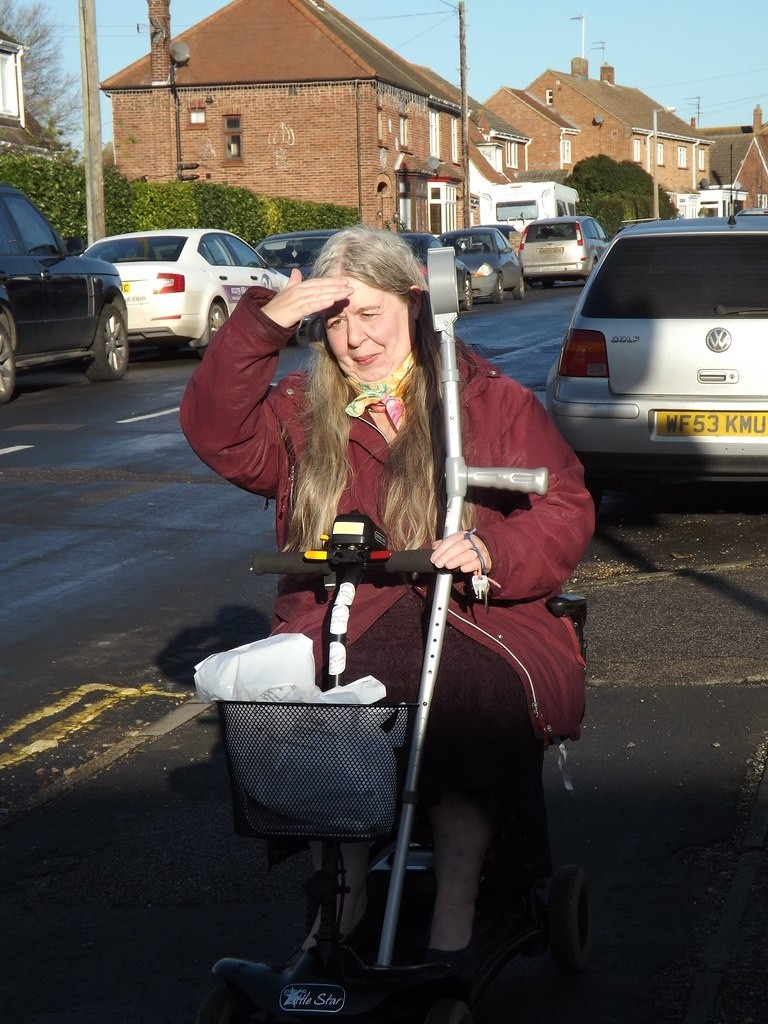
[180,220,595,987]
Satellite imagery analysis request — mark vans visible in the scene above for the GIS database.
[480,180,580,240]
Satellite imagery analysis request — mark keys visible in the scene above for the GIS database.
[472,573,490,608]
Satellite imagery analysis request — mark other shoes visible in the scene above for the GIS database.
[211,946,331,993]
[402,938,476,1002]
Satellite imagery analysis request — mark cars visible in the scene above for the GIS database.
[545,213,767,489]
[436,227,526,305]
[737,206,768,217]
[250,228,339,344]
[471,223,517,239]
[395,233,474,313]
[518,215,610,286]
[75,230,305,359]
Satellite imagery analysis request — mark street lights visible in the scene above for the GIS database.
[652,105,677,221]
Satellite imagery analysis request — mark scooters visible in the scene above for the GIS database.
[182,511,590,1024]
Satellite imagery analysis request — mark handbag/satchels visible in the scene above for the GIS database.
[192,632,408,832]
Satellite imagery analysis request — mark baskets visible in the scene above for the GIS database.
[214,698,422,844]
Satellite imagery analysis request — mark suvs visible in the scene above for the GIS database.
[0,184,132,406]
